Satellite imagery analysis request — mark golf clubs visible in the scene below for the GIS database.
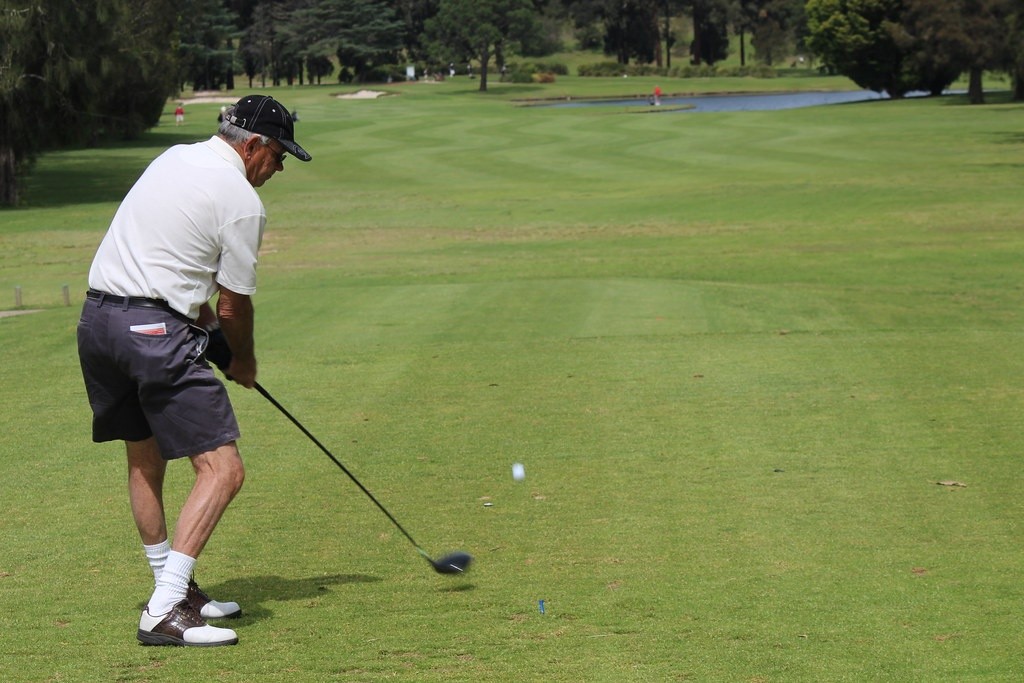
[212,345,477,578]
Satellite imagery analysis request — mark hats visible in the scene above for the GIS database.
[224,95,312,162]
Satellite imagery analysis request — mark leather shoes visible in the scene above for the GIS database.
[137,598,238,646]
[186,569,241,619]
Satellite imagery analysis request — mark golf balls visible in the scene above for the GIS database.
[512,463,525,480]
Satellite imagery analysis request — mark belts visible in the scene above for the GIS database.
[87,291,170,309]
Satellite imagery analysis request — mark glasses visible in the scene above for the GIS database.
[246,137,287,163]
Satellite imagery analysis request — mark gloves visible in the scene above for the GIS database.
[204,320,233,381]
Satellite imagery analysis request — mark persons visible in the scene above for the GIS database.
[653,85,662,106]
[175,103,184,127]
[75,95,314,648]
